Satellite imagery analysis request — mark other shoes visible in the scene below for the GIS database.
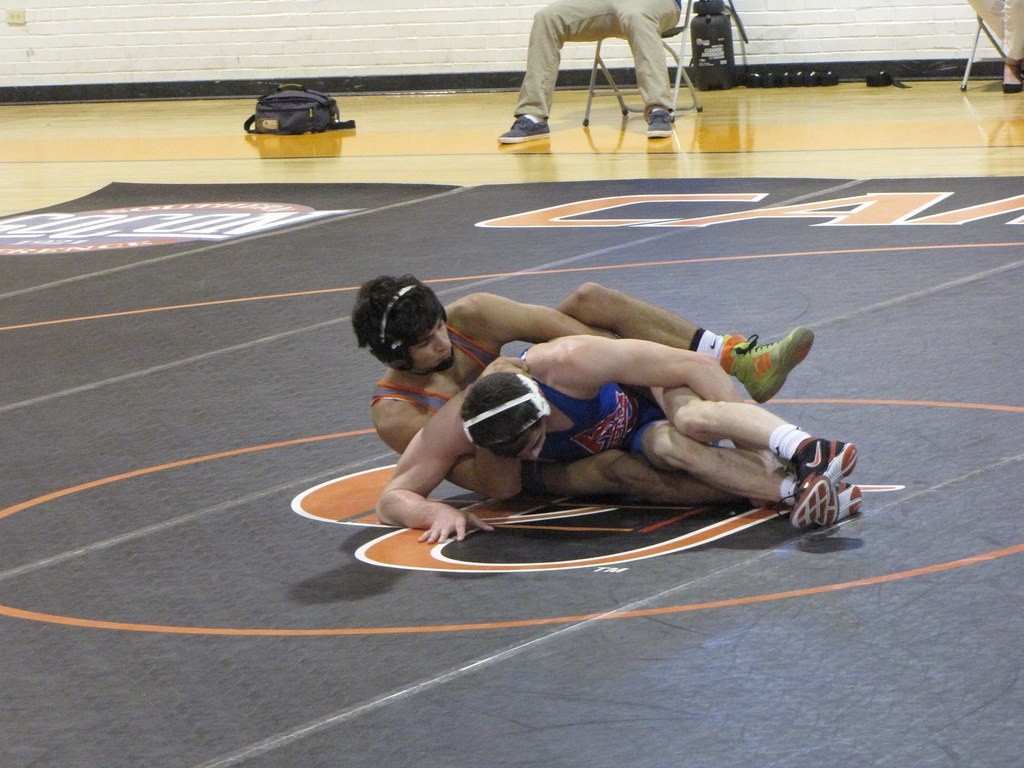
[821,485,865,527]
[498,114,551,145]
[790,437,859,527]
[646,110,676,139]
[725,325,817,405]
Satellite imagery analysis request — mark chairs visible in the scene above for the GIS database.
[962,15,1005,91]
[584,1,702,129]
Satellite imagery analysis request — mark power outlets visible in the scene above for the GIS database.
[6,9,25,23]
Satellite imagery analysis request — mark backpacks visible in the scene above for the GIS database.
[244,83,358,137]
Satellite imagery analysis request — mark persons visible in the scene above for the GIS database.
[966,0,1024,93]
[497,0,682,143]
[375,334,862,544]
[351,274,815,503]
